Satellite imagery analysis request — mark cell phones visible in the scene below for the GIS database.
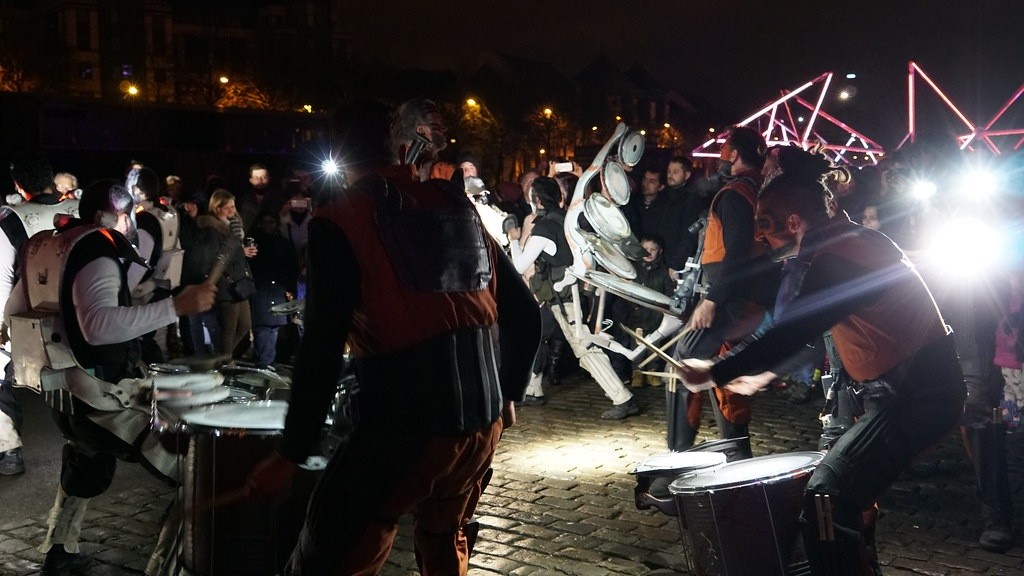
[554,163,573,173]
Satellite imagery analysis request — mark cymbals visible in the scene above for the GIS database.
[170,350,233,371]
[219,364,290,390]
[575,227,617,257]
[269,298,305,316]
[683,436,749,452]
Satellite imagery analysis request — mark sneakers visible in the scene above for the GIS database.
[631,370,643,388]
[645,370,663,387]
[515,395,549,411]
[601,398,641,419]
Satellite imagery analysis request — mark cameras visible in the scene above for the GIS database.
[887,168,911,184]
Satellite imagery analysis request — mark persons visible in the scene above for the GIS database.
[6,180,217,576]
[246,95,543,576]
[650,126,1024,576]
[0,92,706,421]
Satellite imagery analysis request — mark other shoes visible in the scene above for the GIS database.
[0,450,25,475]
[778,381,821,404]
[40,547,91,575]
[979,521,1012,552]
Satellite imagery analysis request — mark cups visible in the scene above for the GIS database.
[245,238,254,247]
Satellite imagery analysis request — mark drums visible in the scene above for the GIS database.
[592,241,637,280]
[147,362,191,377]
[585,192,631,241]
[142,374,225,394]
[627,451,831,576]
[178,398,336,576]
[622,131,646,167]
[156,385,232,406]
[587,269,674,307]
[604,161,630,206]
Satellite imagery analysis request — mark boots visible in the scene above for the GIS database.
[546,338,565,386]
